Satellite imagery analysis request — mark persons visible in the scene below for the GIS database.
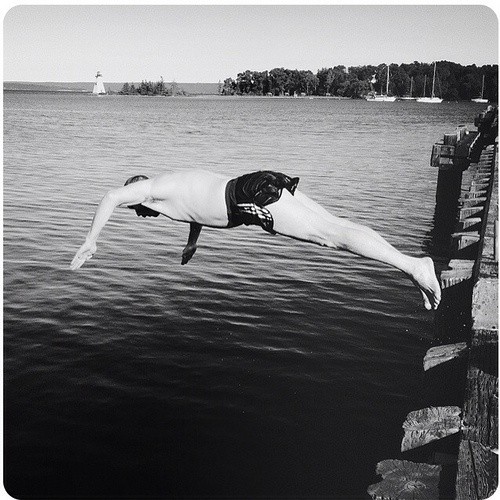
[469,96,499,165]
[67,169,442,311]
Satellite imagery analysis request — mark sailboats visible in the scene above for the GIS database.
[361,61,445,104]
[471,74,488,103]
